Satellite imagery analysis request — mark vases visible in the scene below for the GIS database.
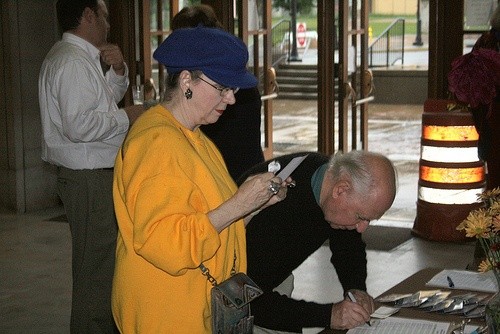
[485,272,500,334]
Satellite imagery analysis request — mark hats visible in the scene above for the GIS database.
[153,27,258,88]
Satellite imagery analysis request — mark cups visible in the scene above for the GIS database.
[131,85,144,105]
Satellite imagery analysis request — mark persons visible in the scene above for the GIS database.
[110,26,297,334]
[37,0,157,334]
[235,150,396,334]
[170,4,265,183]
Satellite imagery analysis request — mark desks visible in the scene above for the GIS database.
[319,268,495,334]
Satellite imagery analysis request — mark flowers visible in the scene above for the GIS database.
[456,185,500,281]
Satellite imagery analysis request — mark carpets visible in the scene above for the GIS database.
[45,214,413,253]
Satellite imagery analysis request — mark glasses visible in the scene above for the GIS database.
[197,76,239,97]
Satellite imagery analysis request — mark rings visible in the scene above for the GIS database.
[268,181,280,194]
[287,180,296,188]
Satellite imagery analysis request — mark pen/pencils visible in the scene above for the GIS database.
[447,275,454,288]
[346,291,371,327]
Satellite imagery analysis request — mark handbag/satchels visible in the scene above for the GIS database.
[211,272,263,334]
